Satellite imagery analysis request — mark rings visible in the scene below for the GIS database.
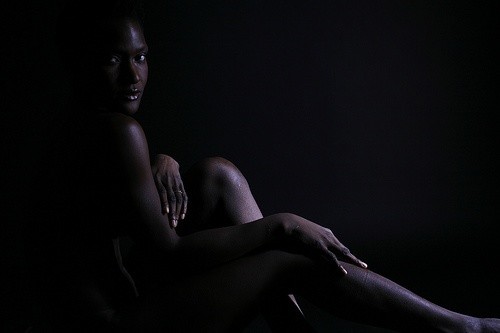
[175,190,183,195]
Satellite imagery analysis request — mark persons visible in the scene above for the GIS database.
[43,0,500,333]
